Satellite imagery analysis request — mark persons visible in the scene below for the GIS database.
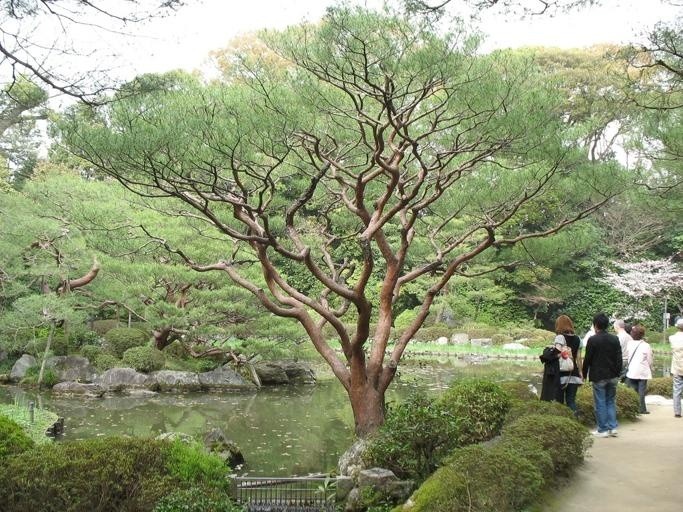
[612,319,634,386]
[624,325,654,415]
[545,313,583,419]
[667,318,682,418]
[581,312,624,439]
[623,323,633,337]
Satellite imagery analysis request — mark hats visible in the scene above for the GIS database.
[613,319,625,328]
[675,318,683,330]
[630,325,645,338]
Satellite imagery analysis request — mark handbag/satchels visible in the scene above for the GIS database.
[558,346,575,372]
[620,364,628,384]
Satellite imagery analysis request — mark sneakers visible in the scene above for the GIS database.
[610,430,619,438]
[590,429,608,438]
[673,414,683,419]
[638,411,650,415]
